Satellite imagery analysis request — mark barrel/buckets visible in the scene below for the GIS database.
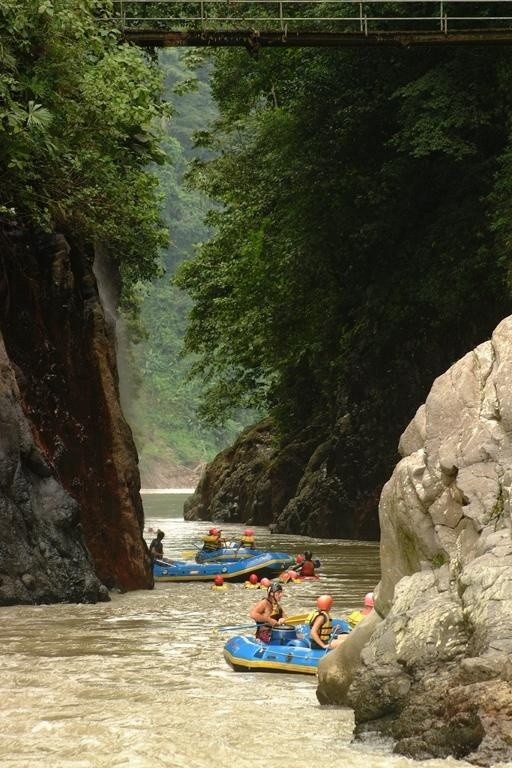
[272,623,298,646]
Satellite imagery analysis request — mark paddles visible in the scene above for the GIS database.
[155,551,193,557]
[216,613,308,631]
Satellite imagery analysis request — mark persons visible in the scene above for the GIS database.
[307,594,350,650]
[346,592,377,631]
[194,528,224,565]
[291,550,315,577]
[239,530,256,550]
[249,584,289,644]
[148,530,176,568]
[209,569,302,590]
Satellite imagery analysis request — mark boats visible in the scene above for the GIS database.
[223,618,353,674]
[153,535,295,581]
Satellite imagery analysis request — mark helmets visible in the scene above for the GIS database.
[266,582,283,595]
[317,593,334,610]
[244,529,253,536]
[214,575,224,585]
[363,591,376,607]
[296,549,313,563]
[208,527,218,535]
[249,573,259,585]
[260,577,271,586]
